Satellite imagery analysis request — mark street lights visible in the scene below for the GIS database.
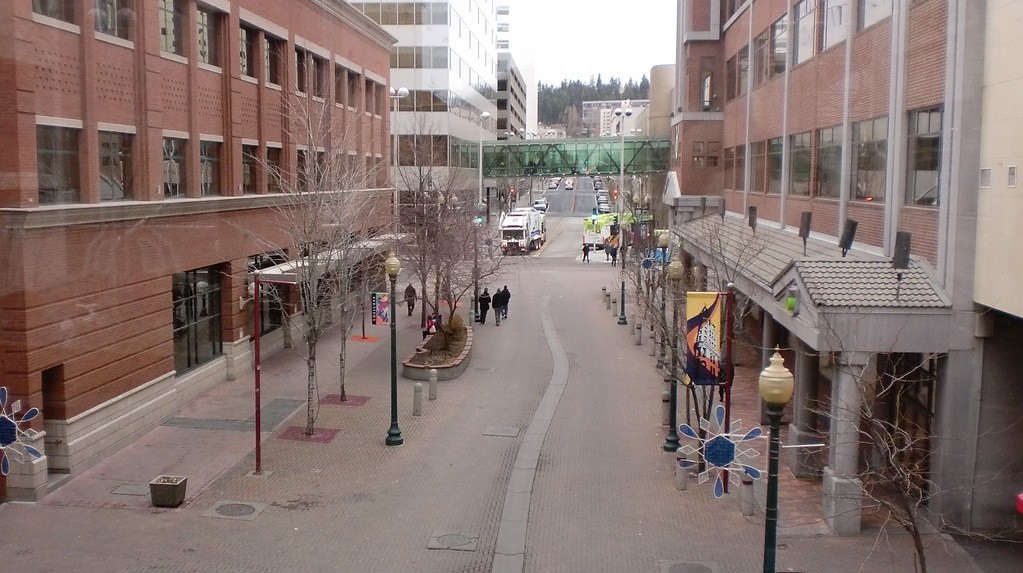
[618,217,629,325]
[418,194,457,327]
[383,249,405,446]
[655,230,670,370]
[663,251,685,453]
[759,345,795,573]
[389,86,409,255]
[471,216,481,324]
[475,111,491,216]
[613,107,633,216]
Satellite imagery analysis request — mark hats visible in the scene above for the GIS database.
[409,282,412,285]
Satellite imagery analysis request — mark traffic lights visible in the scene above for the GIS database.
[613,215,618,224]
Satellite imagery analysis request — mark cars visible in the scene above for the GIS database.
[549,179,573,190]
[597,195,608,205]
[593,176,601,183]
[596,190,608,198]
[598,203,611,214]
[594,181,603,190]
[533,200,548,212]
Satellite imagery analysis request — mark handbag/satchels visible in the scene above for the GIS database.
[429,325,436,333]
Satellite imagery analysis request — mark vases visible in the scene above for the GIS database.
[148,474,188,508]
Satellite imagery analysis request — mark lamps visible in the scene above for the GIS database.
[748,206,757,238]
[718,199,725,227]
[700,197,707,228]
[240,282,262,310]
[893,231,912,301]
[797,212,812,256]
[839,218,858,258]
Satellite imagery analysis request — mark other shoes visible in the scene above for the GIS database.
[408,313,412,316]
[497,324,499,326]
[502,313,507,319]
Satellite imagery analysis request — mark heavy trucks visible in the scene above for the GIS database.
[494,207,541,256]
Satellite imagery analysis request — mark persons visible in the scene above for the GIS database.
[612,246,617,267]
[582,242,590,264]
[501,285,511,319]
[492,287,504,327]
[479,287,491,325]
[422,313,439,341]
[605,242,612,261]
[404,283,418,317]
